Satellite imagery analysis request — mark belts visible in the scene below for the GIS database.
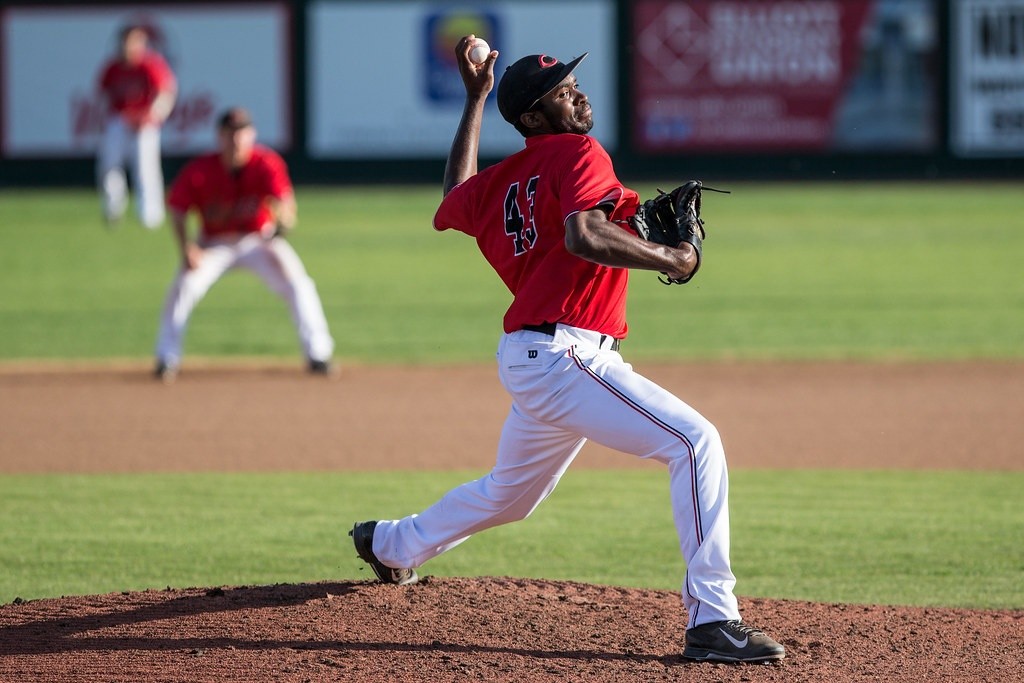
[521,320,621,351]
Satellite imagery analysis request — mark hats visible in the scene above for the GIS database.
[218,110,252,130]
[496,51,589,125]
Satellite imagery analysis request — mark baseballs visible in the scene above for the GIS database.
[468,38,491,66]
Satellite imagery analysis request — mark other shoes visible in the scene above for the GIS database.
[307,360,334,374]
[153,363,181,379]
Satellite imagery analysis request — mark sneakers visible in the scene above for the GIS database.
[681,620,785,663]
[348,521,419,586]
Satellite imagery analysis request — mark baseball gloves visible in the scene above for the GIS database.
[630,180,706,284]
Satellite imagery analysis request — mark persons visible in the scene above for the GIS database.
[153,106,337,382]
[349,33,788,664]
[91,21,179,233]
